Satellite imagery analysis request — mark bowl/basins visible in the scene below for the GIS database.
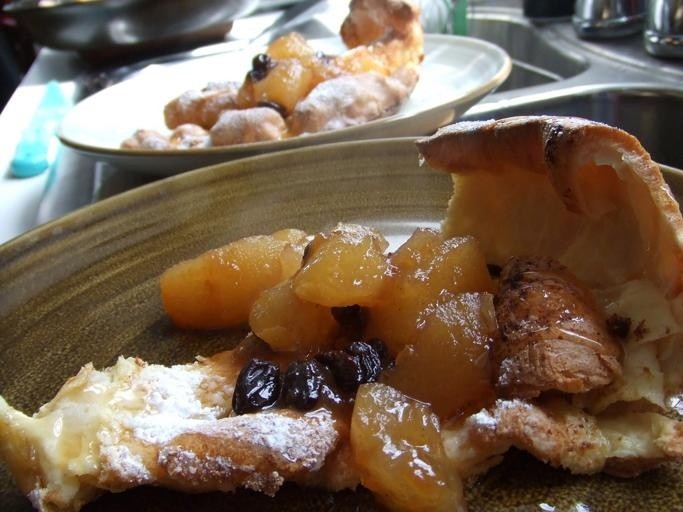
[0,0,260,51]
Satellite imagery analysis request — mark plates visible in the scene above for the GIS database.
[53,33,514,178]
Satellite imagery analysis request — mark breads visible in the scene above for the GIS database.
[122,1,425,150]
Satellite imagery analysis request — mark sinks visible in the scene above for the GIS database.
[0,83,683,231]
[23,2,592,115]
